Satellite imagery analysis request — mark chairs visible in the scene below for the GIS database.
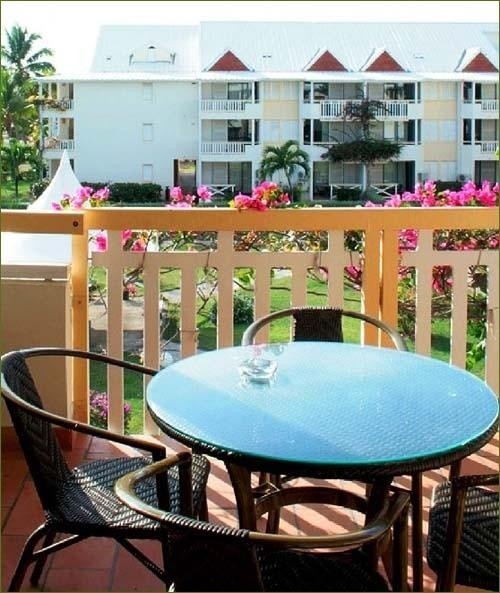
[1,345,211,591]
[115,452,411,592]
[426,456,500,592]
[239,308,413,533]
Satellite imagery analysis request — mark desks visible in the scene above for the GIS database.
[146,342,497,592]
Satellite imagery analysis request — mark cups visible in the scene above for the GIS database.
[238,344,284,388]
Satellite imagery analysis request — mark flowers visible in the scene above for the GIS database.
[49,181,499,298]
